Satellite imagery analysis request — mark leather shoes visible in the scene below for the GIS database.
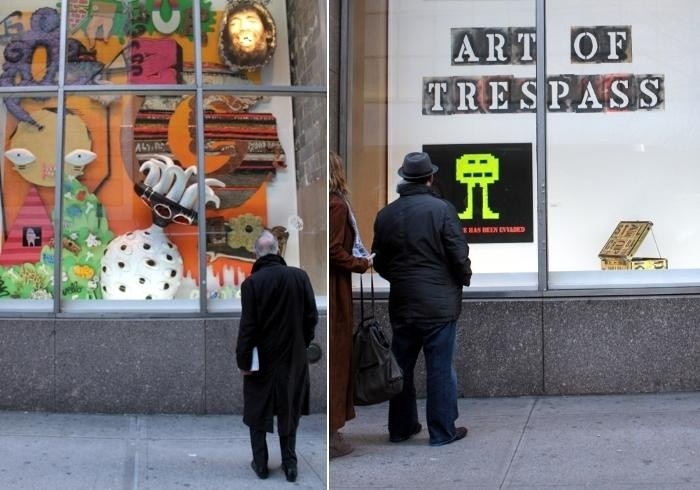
[250,459,270,479]
[452,426,468,442]
[281,462,298,482]
[390,423,422,443]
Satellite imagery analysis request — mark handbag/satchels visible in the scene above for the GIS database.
[350,315,405,408]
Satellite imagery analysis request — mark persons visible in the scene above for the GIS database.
[369,151,473,445]
[329,148,375,461]
[236,230,319,483]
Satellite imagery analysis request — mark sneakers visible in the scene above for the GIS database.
[329,436,353,460]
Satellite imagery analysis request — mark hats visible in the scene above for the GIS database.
[395,151,440,180]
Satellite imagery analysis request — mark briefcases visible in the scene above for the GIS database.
[598,218,668,272]
